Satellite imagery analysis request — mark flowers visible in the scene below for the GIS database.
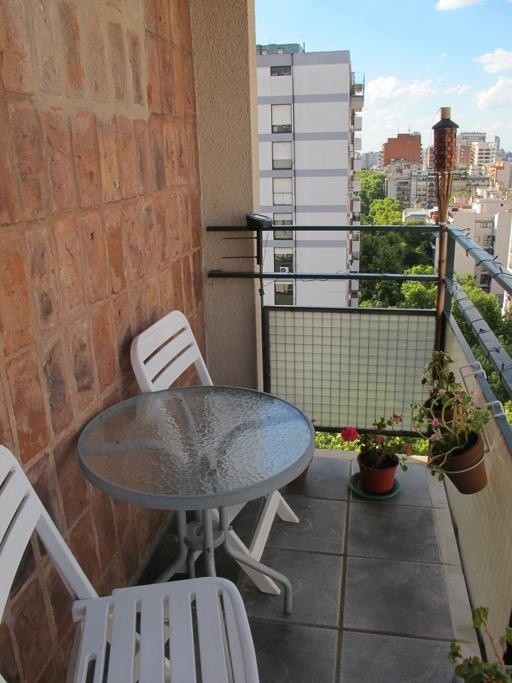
[340,411,414,472]
[405,386,491,482]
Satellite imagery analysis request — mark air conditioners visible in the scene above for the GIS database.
[280,266,289,273]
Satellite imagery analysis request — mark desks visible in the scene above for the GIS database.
[76,387,315,616]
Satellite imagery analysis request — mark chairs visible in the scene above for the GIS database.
[129,309,300,595]
[0,444,260,683]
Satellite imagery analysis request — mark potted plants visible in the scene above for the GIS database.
[420,352,467,438]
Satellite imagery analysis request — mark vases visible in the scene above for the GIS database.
[431,430,488,495]
[355,452,400,497]
